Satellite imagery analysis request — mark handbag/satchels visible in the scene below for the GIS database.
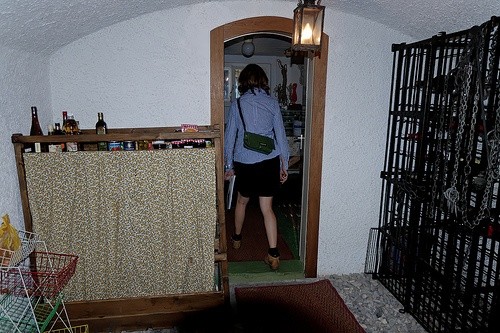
[243,131,275,155]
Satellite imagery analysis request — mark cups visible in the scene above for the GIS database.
[293,128,301,137]
[293,120,303,128]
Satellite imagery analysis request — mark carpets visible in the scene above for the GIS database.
[226,207,295,263]
[234,278,368,333]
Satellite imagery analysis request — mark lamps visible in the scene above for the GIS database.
[241,34,255,56]
[292,0,326,52]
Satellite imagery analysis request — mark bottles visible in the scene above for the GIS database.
[30,106,45,153]
[96,112,110,149]
[53,112,84,153]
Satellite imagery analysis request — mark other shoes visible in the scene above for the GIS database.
[230,233,241,250]
[262,249,281,270]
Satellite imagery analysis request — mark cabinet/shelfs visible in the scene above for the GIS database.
[0,227,89,333]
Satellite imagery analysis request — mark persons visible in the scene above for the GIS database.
[225,63,290,271]
